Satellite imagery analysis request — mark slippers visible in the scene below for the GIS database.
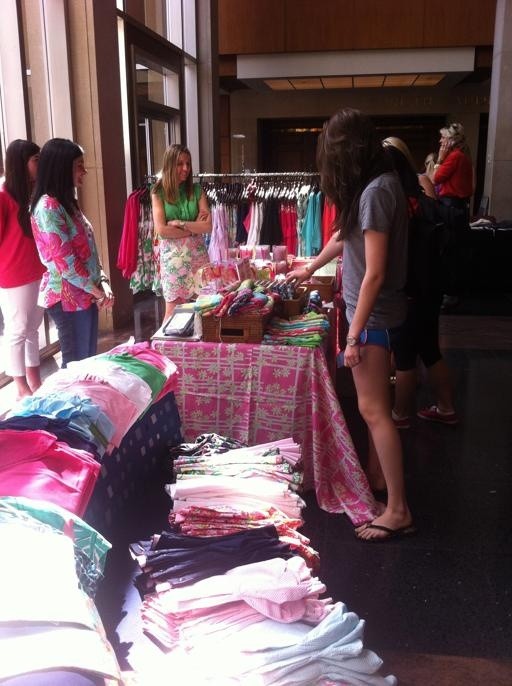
[355,519,415,542]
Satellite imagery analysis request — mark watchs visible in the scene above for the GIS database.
[344,334,364,347]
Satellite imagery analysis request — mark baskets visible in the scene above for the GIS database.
[282,288,310,321]
[202,311,271,343]
[297,276,335,302]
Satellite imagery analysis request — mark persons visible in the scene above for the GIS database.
[34,137,118,370]
[380,135,439,200]
[313,106,414,541]
[150,142,214,329]
[283,144,460,428]
[419,150,440,194]
[1,137,50,399]
[430,122,475,310]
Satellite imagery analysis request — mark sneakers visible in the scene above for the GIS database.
[389,410,412,428]
[416,406,457,424]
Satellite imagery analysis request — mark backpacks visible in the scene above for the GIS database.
[409,206,451,271]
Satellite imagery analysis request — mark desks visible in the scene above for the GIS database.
[154,336,378,527]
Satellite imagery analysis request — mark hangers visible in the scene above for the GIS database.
[137,170,319,203]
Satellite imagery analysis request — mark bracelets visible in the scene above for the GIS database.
[179,220,186,230]
[433,163,441,169]
[304,262,315,276]
[96,292,107,305]
